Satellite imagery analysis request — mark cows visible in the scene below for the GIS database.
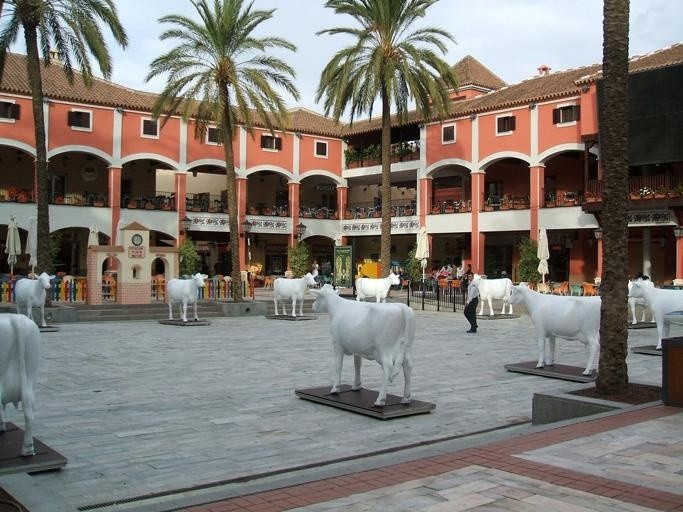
[627,297,656,325]
[271,271,318,318]
[309,282,418,407]
[508,282,602,377]
[14,271,56,327]
[353,272,402,304]
[629,278,683,351]
[0,312,45,456]
[163,272,209,323]
[473,272,515,318]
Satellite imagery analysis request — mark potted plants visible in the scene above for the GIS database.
[432,182,683,214]
[343,139,420,169]
[12,194,228,213]
[247,206,415,219]
[519,234,542,292]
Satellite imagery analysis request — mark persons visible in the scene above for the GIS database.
[464,272,481,332]
[368,203,380,217]
[461,264,471,291]
[354,206,362,219]
[312,259,320,277]
[202,262,209,274]
[436,263,464,280]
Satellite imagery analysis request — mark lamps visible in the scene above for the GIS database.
[294,222,306,240]
[593,228,603,240]
[179,216,192,235]
[672,226,683,239]
[239,218,252,238]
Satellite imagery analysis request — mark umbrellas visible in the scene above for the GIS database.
[537,225,551,284]
[25,213,37,280]
[4,215,24,275]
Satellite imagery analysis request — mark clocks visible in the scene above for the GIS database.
[132,234,142,245]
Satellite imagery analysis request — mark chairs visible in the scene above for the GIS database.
[399,275,410,291]
[553,281,598,296]
[439,278,462,296]
